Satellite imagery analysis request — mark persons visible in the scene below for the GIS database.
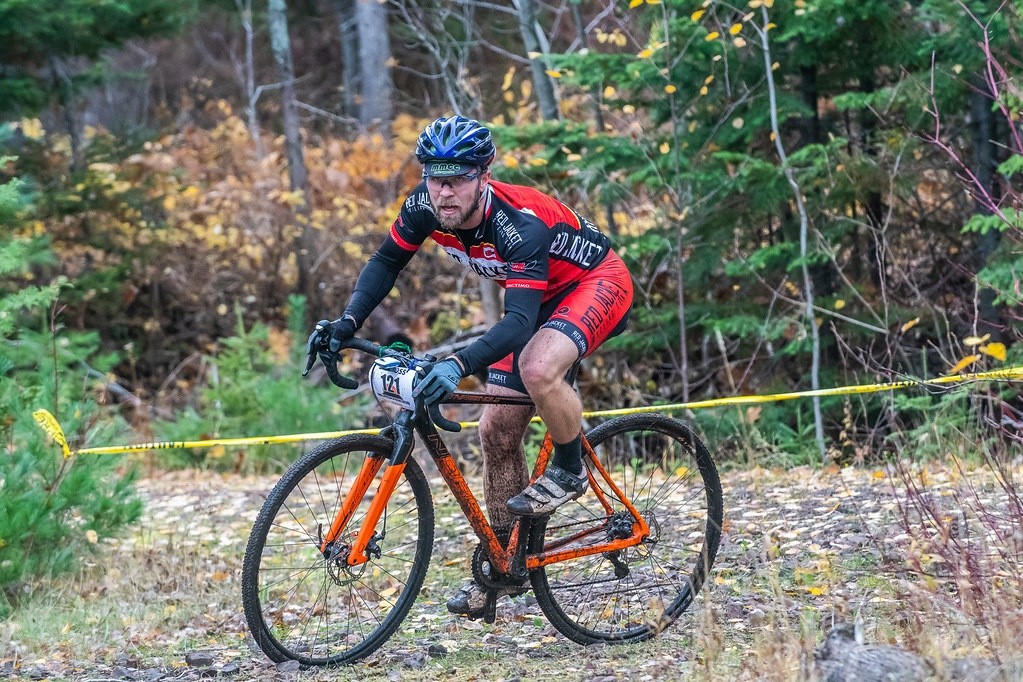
[307,115,632,614]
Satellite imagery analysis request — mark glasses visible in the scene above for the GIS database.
[425,169,488,193]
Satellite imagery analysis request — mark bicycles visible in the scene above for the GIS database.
[244,319,722,670]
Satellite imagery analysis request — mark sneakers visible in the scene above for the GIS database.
[446,565,531,614]
[506,463,589,515]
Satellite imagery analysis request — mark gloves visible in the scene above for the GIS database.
[412,356,466,406]
[306,315,356,362]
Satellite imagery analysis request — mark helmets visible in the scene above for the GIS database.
[415,115,495,176]
